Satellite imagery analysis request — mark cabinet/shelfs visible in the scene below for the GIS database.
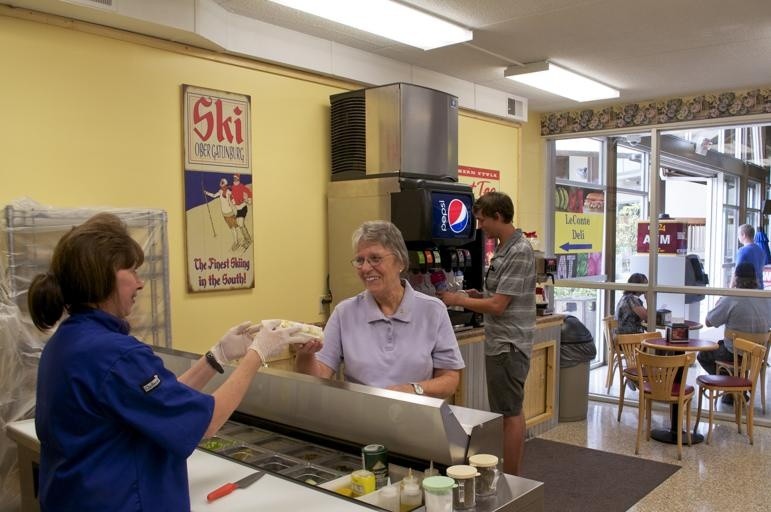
[423,314,564,440]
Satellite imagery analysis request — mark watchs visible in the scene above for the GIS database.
[410,381,425,395]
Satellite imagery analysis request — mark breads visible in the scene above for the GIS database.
[554,186,583,211]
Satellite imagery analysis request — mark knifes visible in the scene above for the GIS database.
[207,470,268,502]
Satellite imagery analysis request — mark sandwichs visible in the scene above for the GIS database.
[277,319,324,344]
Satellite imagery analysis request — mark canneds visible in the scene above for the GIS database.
[447,465,477,510]
[362,443,388,490]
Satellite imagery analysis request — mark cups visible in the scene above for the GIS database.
[422,476,459,512]
[430,271,449,293]
[446,464,482,510]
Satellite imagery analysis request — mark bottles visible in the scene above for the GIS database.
[400,475,423,512]
[379,477,400,512]
[399,468,420,496]
[421,460,442,505]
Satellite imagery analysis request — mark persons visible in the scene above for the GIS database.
[28,213,309,512]
[729,223,766,290]
[616,272,669,357]
[434,191,537,477]
[697,261,771,405]
[295,219,467,400]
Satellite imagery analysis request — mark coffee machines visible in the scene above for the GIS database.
[531,250,554,317]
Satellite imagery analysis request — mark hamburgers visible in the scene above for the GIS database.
[584,193,603,213]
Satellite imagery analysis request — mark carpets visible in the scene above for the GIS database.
[520,437,682,512]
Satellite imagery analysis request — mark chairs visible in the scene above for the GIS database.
[715,326,771,410]
[693,336,766,446]
[633,345,694,459]
[613,331,660,421]
[602,315,632,394]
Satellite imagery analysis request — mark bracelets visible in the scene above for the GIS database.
[206,349,226,375]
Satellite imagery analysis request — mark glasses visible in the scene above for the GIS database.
[351,253,396,269]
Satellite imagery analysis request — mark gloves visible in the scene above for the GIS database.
[210,321,262,370]
[248,319,309,367]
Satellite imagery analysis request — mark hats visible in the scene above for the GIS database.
[734,261,757,279]
